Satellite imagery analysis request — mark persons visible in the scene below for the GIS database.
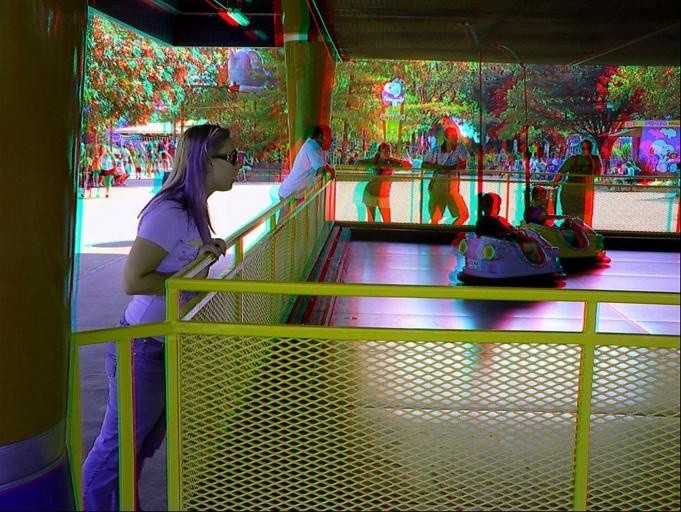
[80,122,239,512]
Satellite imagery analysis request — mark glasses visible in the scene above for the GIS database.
[211,150,239,166]
